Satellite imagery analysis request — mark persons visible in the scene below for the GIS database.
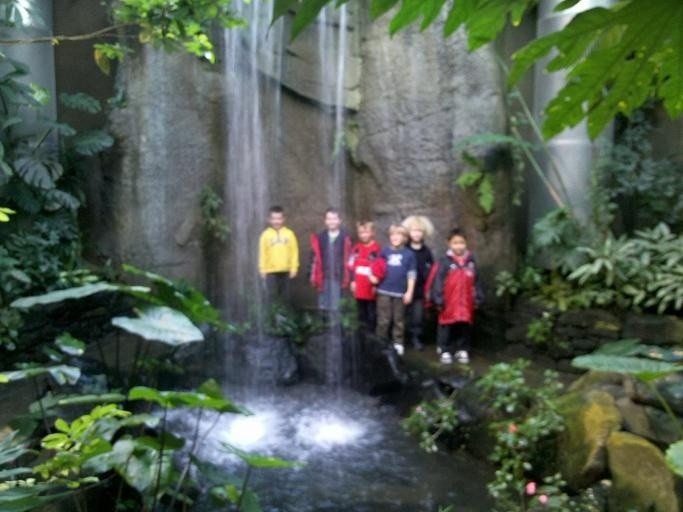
[421,225,485,366]
[306,208,351,313]
[397,213,436,342]
[376,223,419,356]
[256,205,299,309]
[347,215,387,333]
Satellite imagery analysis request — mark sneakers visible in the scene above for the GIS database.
[435,346,473,367]
